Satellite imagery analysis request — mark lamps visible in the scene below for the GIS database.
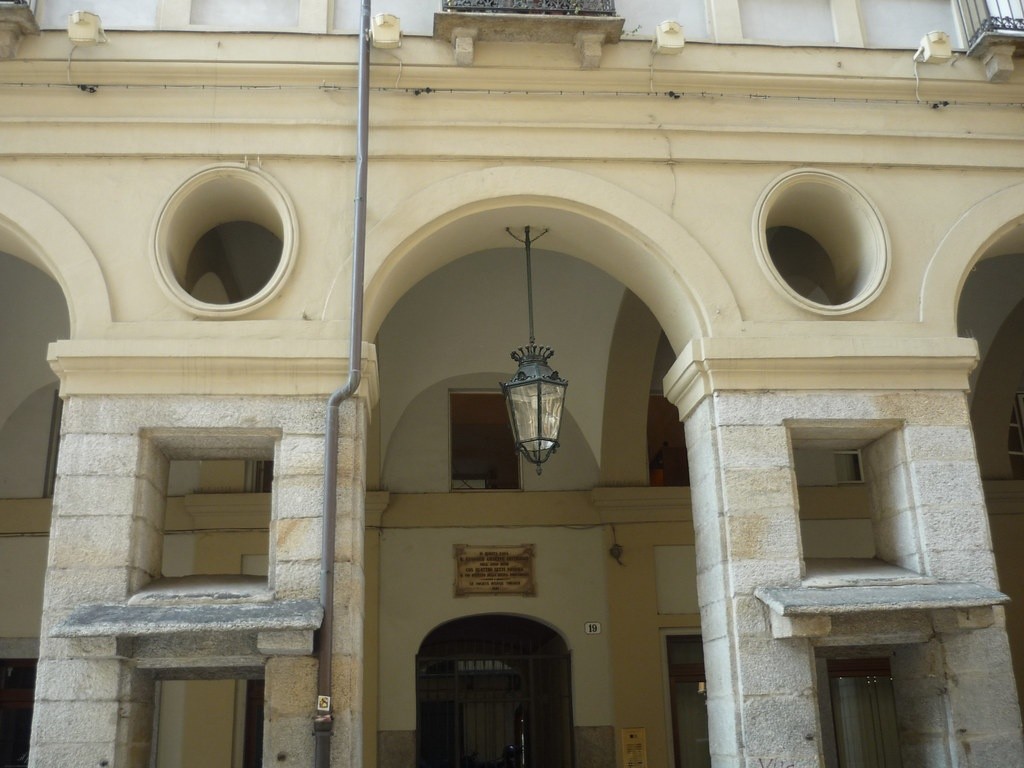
[914,31,952,63]
[655,21,685,55]
[68,10,108,46]
[369,13,403,50]
[498,226,566,474]
